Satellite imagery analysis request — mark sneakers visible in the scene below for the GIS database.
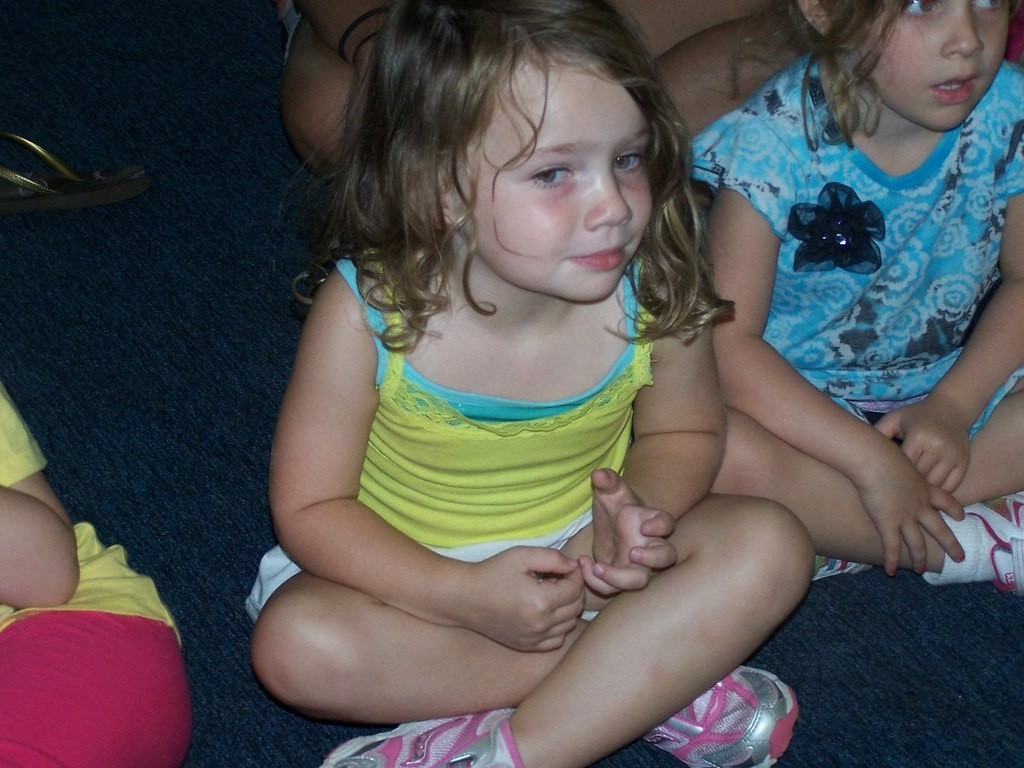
[580,610,797,768]
[811,553,874,580]
[316,707,524,768]
[964,491,1023,596]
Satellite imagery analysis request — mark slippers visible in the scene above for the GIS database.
[0,130,149,212]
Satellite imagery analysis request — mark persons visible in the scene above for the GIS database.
[244,0,815,768]
[282,0,801,185]
[0,382,194,768]
[690,0,1024,598]
